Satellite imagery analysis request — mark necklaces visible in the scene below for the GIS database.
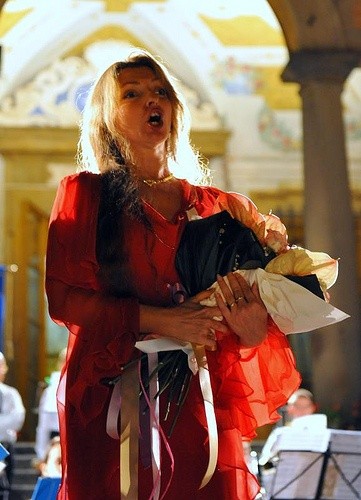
[136,173,174,187]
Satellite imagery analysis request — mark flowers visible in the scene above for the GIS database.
[109,191,351,500]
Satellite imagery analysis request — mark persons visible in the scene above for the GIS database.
[264,388,317,451]
[43,55,301,500]
[0,352,24,484]
[34,349,67,460]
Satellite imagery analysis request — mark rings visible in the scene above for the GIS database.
[208,329,213,338]
[230,303,235,307]
[236,296,244,302]
